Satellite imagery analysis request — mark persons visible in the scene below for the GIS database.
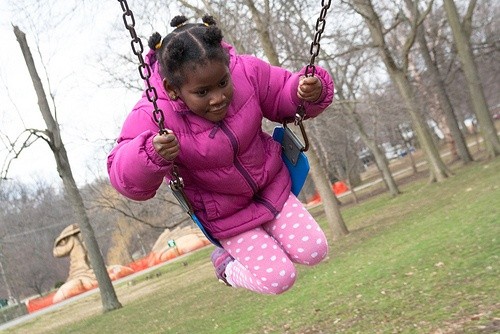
[105,13,336,297]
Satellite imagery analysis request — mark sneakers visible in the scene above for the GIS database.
[212,248,232,288]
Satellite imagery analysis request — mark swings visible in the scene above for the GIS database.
[119,0,332,250]
